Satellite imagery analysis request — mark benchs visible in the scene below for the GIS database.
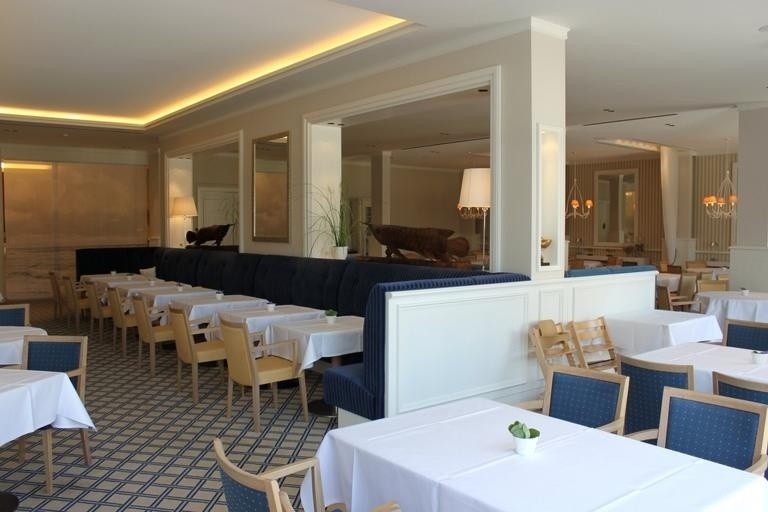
[564,264,657,367]
[76,247,490,368]
[321,267,532,428]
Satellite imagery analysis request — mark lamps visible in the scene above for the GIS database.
[703,139,737,219]
[457,167,494,267]
[565,152,593,219]
[172,196,198,247]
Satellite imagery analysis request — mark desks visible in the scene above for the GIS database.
[169,295,269,381]
[205,304,325,399]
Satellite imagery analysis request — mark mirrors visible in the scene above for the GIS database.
[593,167,640,248]
[252,131,289,242]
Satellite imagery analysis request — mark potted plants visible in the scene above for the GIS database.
[266,302,276,312]
[214,292,224,300]
[306,183,357,258]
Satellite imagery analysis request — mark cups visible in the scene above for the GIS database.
[125,275,132,280]
[508,422,541,455]
[176,285,183,293]
[109,270,117,276]
[266,303,275,312]
[148,280,155,286]
[740,289,749,297]
[215,293,224,301]
[752,350,764,365]
[325,315,338,326]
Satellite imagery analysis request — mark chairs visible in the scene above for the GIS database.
[47,271,136,357]
[168,303,229,404]
[0,303,30,326]
[213,436,402,512]
[129,295,181,374]
[218,313,308,434]
[656,260,731,314]
[17,335,93,494]
[510,278,768,480]
